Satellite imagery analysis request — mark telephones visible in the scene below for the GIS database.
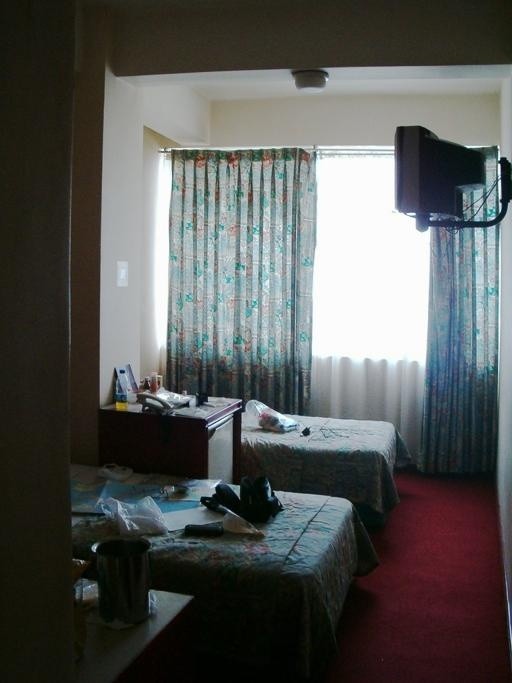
[136,391,178,418]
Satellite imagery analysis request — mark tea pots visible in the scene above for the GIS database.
[91,535,152,625]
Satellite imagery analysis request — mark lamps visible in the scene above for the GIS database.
[291,70,329,93]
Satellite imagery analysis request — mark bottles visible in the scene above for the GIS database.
[142,372,163,394]
[114,369,129,411]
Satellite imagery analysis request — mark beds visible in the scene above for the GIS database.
[69,462,381,683]
[241,411,411,523]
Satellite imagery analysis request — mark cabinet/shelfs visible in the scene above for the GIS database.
[69,581,201,683]
[99,389,244,485]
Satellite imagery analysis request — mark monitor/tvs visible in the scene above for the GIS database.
[395,125,487,219]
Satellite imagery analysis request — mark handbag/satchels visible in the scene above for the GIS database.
[245,399,298,432]
[240,476,285,525]
[199,484,240,514]
